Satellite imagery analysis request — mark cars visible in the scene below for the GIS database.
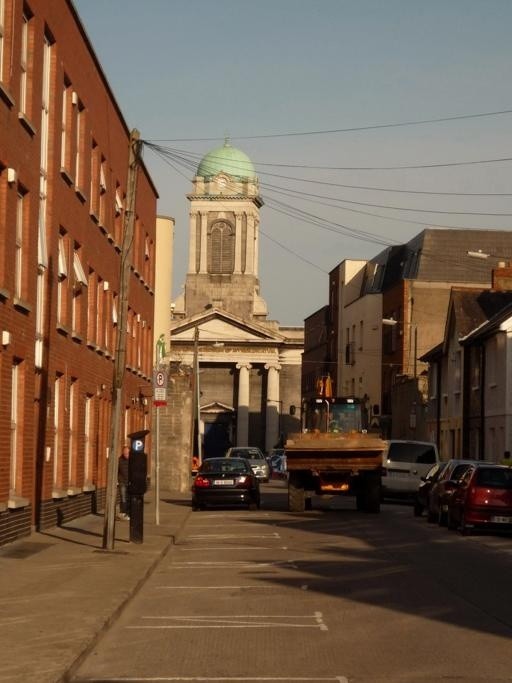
[227,446,270,484]
[192,456,262,510]
[429,458,492,528]
[413,460,446,519]
[438,463,512,538]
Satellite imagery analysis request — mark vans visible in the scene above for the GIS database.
[379,438,441,504]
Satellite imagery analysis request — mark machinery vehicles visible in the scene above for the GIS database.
[282,396,388,514]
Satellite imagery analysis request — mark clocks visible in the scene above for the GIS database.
[216,177,227,188]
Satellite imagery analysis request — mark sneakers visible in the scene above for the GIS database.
[119,513,130,521]
